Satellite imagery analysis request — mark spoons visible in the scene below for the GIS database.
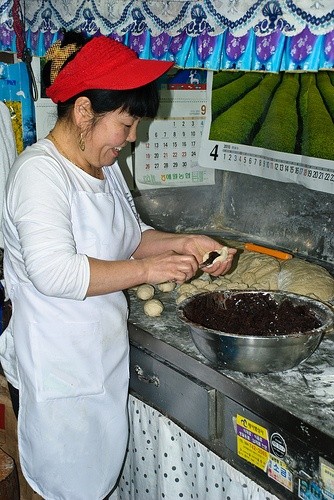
[198,261,211,269]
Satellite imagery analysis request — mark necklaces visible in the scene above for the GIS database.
[49,129,101,180]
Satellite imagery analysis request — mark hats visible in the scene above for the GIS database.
[46,35,176,104]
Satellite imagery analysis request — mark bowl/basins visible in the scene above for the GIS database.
[176,289,334,374]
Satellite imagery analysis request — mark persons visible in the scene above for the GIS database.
[0,34,237,500]
